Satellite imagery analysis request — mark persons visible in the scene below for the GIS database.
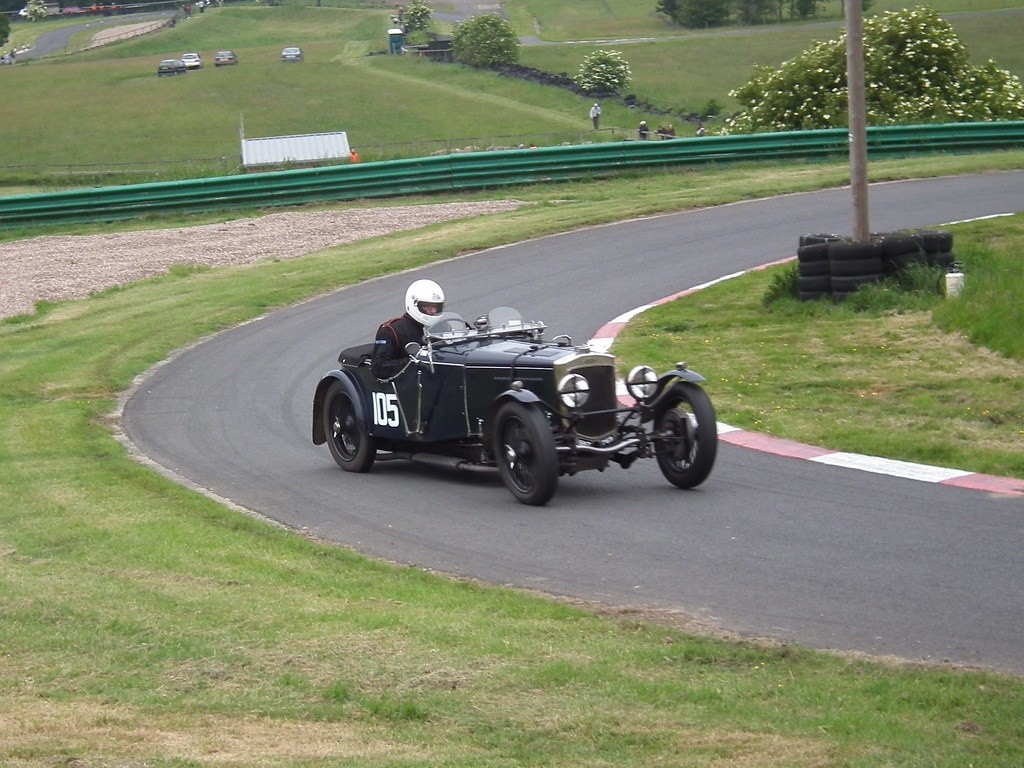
[347,149,359,163]
[369,279,446,378]
[183,0,211,18]
[590,102,602,129]
[637,121,706,140]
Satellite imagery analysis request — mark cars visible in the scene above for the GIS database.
[313,306,717,505]
[64,7,88,16]
[180,52,203,69]
[215,50,240,67]
[281,48,304,63]
[18,8,32,18]
[157,59,188,78]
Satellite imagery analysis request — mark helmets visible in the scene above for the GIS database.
[405,278,445,326]
